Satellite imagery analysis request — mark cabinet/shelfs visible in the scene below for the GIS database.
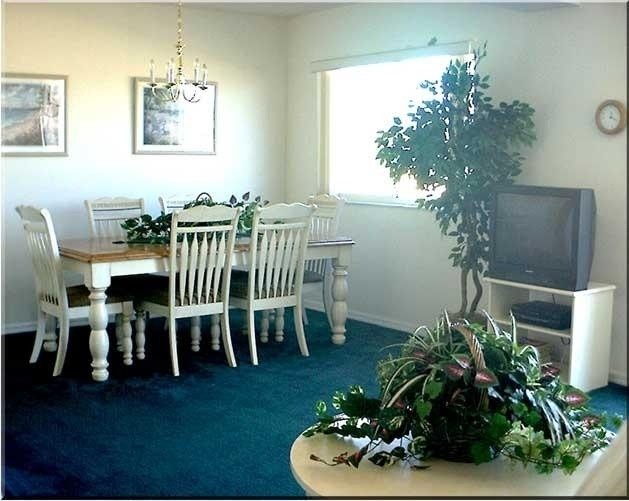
[481,277,617,396]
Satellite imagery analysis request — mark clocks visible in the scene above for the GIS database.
[593,100,627,137]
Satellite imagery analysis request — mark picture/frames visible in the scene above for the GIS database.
[132,76,219,157]
[1,70,70,159]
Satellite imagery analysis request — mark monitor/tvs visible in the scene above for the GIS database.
[487,183,597,293]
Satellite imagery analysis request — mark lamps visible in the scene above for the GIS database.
[148,2,208,104]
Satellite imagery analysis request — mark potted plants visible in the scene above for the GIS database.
[373,37,537,330]
[299,309,625,480]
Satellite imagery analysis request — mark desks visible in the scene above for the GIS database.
[289,412,616,495]
